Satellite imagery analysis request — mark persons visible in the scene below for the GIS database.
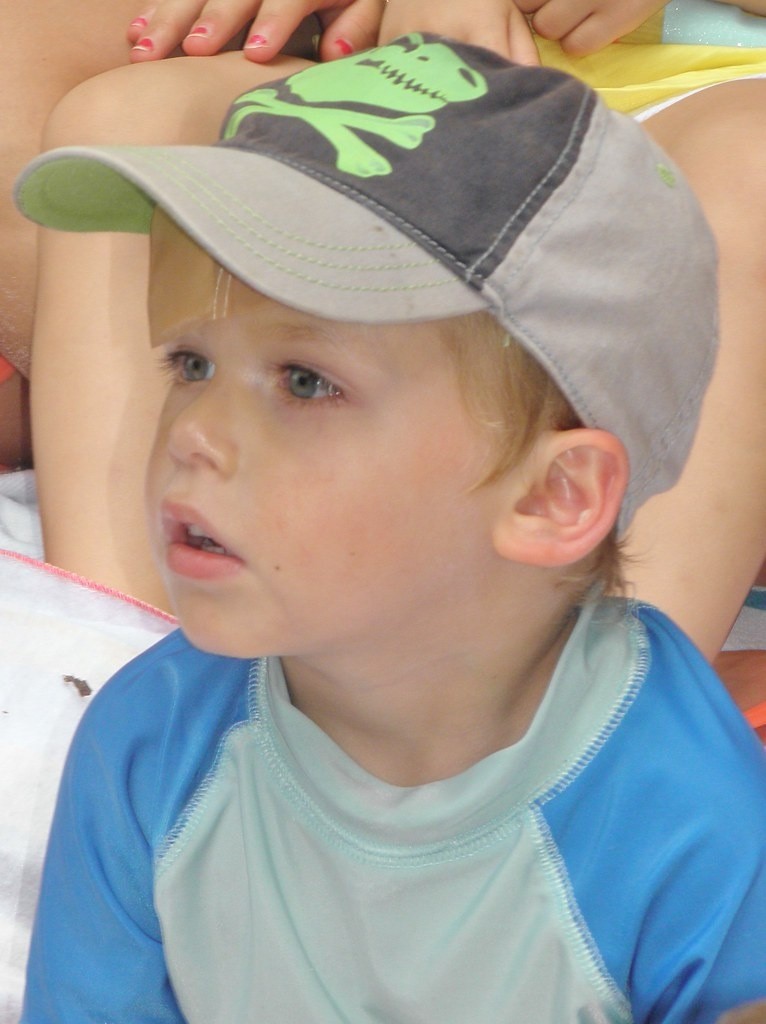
[30,1,765,664]
[15,28,766,1024]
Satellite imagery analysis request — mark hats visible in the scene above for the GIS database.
[13,30,721,542]
